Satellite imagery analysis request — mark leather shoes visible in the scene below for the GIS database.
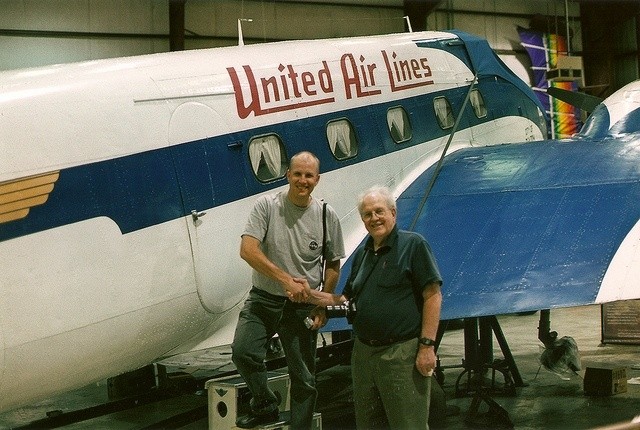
[237,407,280,428]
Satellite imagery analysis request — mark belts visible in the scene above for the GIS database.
[357,330,421,347]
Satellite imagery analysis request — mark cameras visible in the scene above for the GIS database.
[325,298,358,324]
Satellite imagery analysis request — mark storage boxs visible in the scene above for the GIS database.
[584,364,628,397]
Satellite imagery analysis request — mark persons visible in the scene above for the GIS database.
[231,149,347,429]
[292,185,446,430]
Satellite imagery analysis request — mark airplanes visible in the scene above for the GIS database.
[0,16,640,430]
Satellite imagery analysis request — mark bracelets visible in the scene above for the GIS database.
[417,337,436,348]
